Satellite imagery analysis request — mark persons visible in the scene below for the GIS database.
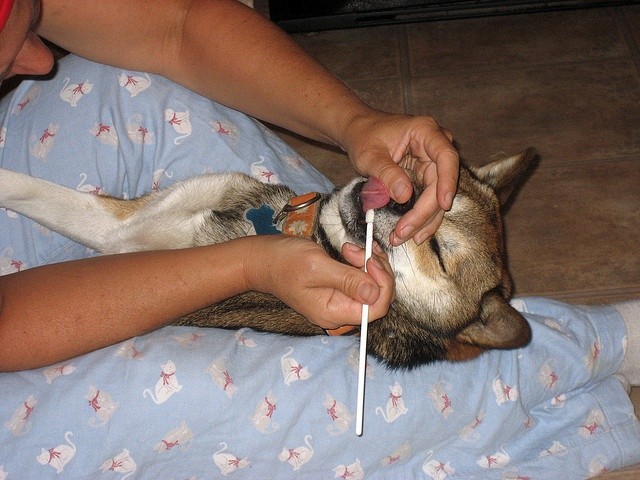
[0,0,460,373]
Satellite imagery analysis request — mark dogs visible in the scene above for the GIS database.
[0,142,548,374]
